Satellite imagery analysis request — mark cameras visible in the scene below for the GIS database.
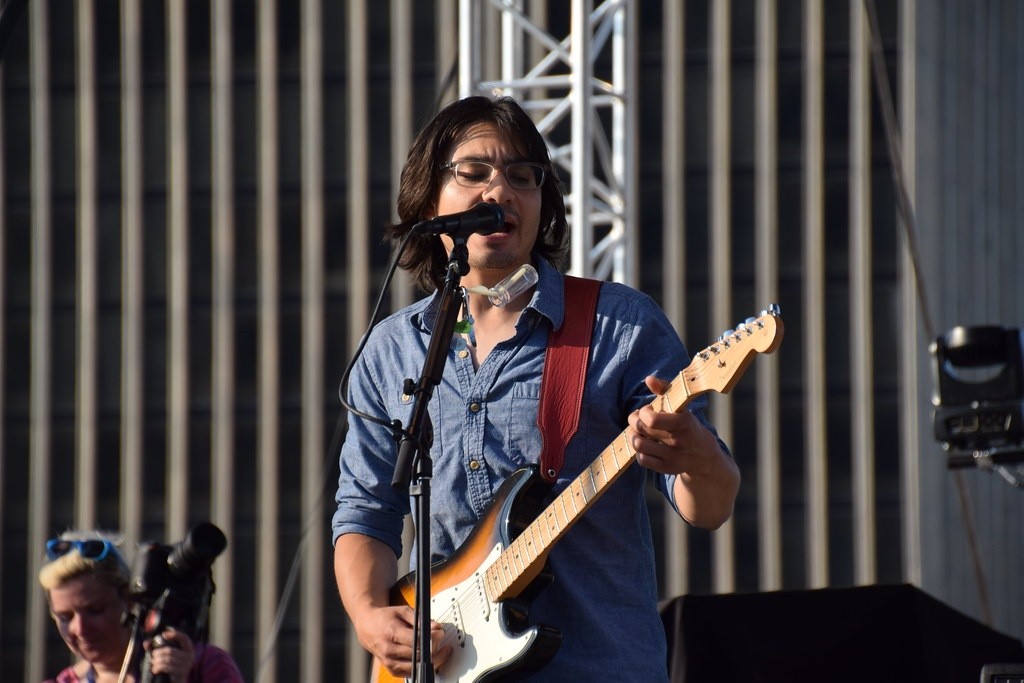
[131,523,228,632]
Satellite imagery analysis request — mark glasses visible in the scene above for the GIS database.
[45,539,133,579]
[437,159,547,191]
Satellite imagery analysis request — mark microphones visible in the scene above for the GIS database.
[415,202,506,235]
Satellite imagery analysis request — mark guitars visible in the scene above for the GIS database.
[367,303,786,683]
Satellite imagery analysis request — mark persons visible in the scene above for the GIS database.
[328,89,742,683]
[41,539,244,683]
